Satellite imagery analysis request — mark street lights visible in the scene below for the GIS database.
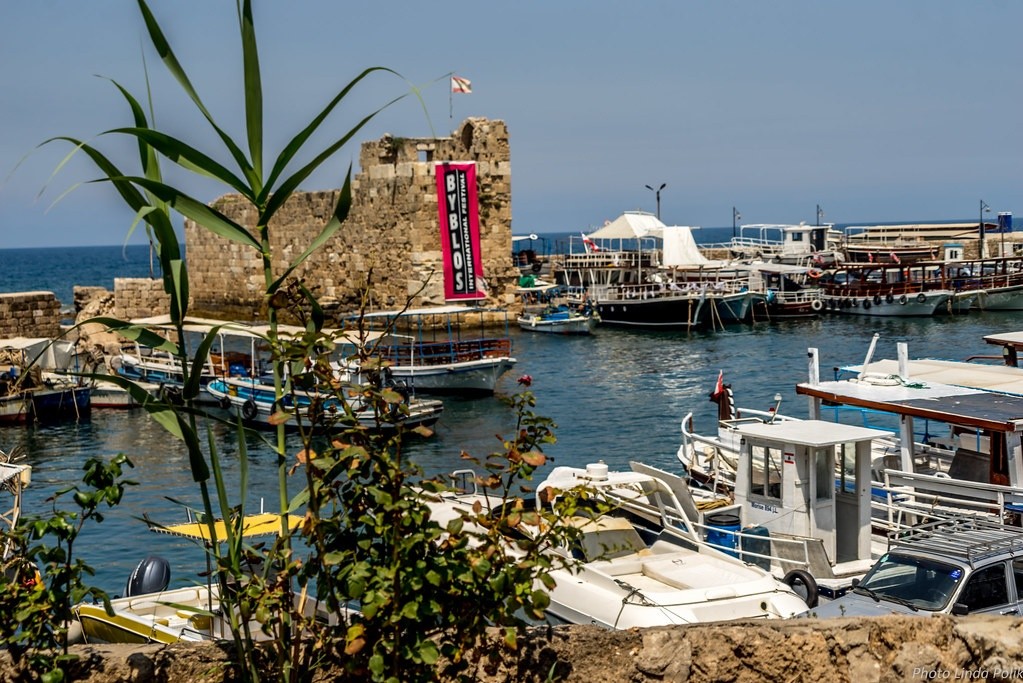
[645,183,665,221]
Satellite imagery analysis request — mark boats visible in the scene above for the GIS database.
[204,324,446,432]
[354,468,809,636]
[676,345,1023,525]
[0,338,160,421]
[553,209,1023,326]
[532,416,938,607]
[74,496,364,647]
[516,285,589,334]
[108,315,231,390]
[342,305,517,397]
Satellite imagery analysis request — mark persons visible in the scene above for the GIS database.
[450,75,473,95]
[526,290,560,309]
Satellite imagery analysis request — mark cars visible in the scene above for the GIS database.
[936,261,1023,280]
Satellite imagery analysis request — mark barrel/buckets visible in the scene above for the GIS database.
[741,525,771,572]
[705,514,741,559]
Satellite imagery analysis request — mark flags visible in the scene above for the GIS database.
[734,209,741,220]
[582,233,599,252]
[818,206,824,217]
[714,371,723,398]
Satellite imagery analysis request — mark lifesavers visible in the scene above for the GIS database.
[916,293,926,303]
[863,299,871,309]
[845,298,850,308]
[858,372,902,386]
[219,396,231,409]
[873,294,882,305]
[837,299,844,308]
[812,300,823,311]
[242,400,258,419]
[851,298,859,307]
[886,293,894,303]
[782,570,819,608]
[898,294,908,305]
[828,299,835,309]
[808,268,820,278]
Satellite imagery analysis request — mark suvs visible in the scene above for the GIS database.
[792,513,1023,626]
